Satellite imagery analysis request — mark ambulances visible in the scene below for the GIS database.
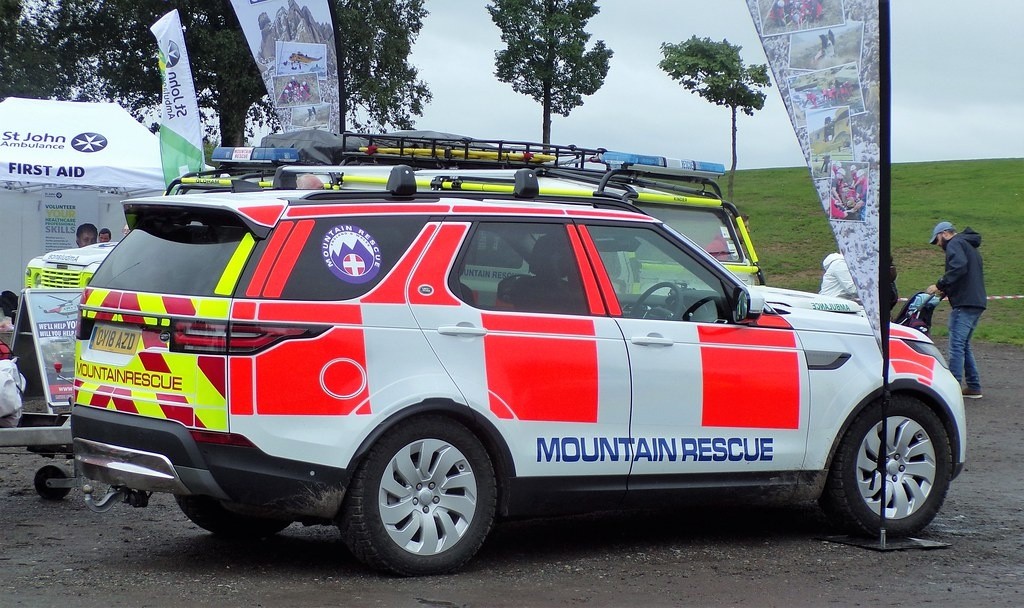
[24,147,300,289]
[283,133,764,288]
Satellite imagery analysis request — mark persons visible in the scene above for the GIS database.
[123,224,131,238]
[928,221,986,400]
[816,250,863,307]
[762,0,879,220]
[75,223,98,249]
[704,211,751,263]
[273,52,330,131]
[99,228,112,243]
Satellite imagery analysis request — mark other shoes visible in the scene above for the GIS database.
[962,389,982,398]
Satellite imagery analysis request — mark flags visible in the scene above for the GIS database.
[151,10,206,195]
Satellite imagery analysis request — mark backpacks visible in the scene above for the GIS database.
[903,313,928,337]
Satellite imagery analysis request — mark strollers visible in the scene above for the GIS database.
[890,289,947,340]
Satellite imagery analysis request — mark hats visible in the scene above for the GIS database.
[850,165,856,171]
[929,222,956,245]
[837,168,846,176]
[857,170,864,178]
[836,161,842,167]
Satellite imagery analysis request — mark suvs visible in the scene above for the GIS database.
[70,187,966,577]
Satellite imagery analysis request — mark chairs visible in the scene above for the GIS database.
[512,231,577,313]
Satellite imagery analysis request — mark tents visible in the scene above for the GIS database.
[0,96,217,335]
